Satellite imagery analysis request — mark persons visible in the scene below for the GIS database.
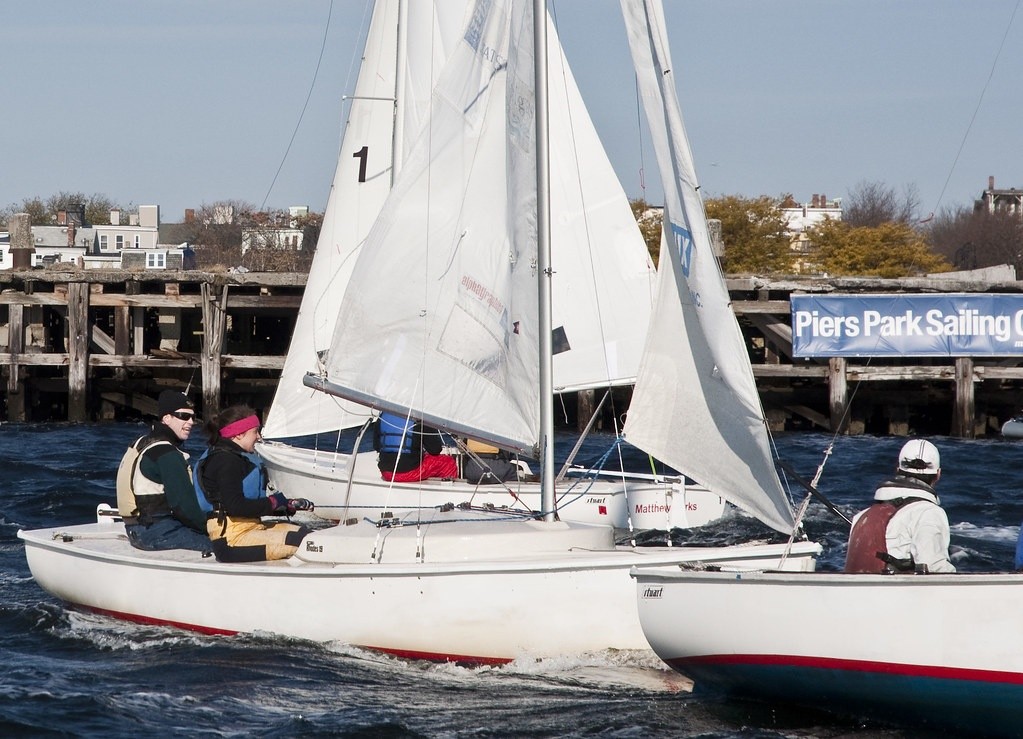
[193,406,320,561]
[118,391,213,551]
[846,438,995,573]
[372,412,458,481]
[461,436,524,482]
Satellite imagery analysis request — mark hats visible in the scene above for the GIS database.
[899,439,940,474]
[159,390,194,420]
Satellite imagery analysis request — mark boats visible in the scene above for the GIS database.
[629,561,1022,737]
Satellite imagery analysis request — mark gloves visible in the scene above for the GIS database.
[288,498,314,512]
[269,491,287,511]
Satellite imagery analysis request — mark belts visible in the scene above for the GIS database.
[468,453,503,460]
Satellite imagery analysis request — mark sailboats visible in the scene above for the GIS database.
[255,0,729,531]
[16,0,825,677]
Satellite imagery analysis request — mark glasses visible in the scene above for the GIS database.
[170,412,196,421]
